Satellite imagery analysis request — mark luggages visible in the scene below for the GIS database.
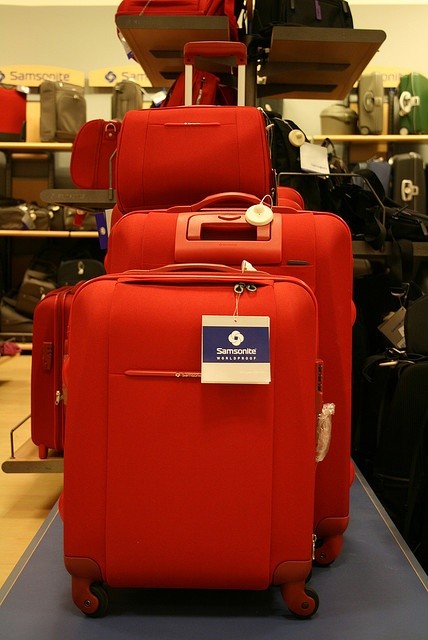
[70,119,122,190]
[61,262,323,616]
[397,72,427,136]
[110,41,306,231]
[112,81,144,118]
[358,73,384,134]
[105,193,357,567]
[388,152,427,215]
[30,282,84,459]
[1,83,29,140]
[39,81,87,143]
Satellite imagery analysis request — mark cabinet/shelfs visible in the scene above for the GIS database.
[1,140,101,355]
[310,130,428,150]
[112,14,232,90]
[263,25,386,105]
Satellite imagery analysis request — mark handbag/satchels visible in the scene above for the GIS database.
[116,0,244,38]
[161,68,219,107]
[251,0,353,99]
[115,106,276,214]
